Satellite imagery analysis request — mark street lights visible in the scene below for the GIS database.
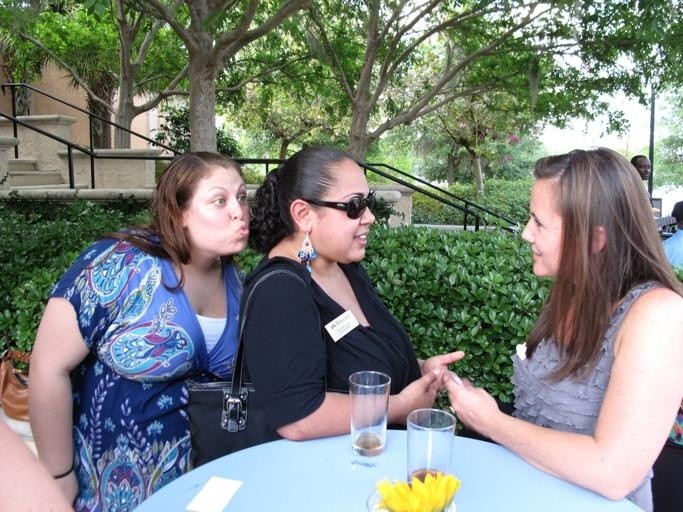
[646,68,666,205]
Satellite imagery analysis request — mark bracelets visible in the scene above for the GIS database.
[51,466,74,479]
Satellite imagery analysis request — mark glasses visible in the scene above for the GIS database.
[302,190,375,218]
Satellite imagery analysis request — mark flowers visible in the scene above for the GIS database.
[373,471,462,512]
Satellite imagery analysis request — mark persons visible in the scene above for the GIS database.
[630,155,652,207]
[26,151,252,512]
[660,201,681,271]
[442,146,682,512]
[234,147,466,447]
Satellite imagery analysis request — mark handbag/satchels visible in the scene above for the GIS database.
[0,350,31,421]
[186,381,352,468]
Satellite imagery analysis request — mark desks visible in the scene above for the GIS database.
[121,428,651,512]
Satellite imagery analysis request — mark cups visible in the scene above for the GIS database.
[348,370,391,466]
[405,408,457,492]
